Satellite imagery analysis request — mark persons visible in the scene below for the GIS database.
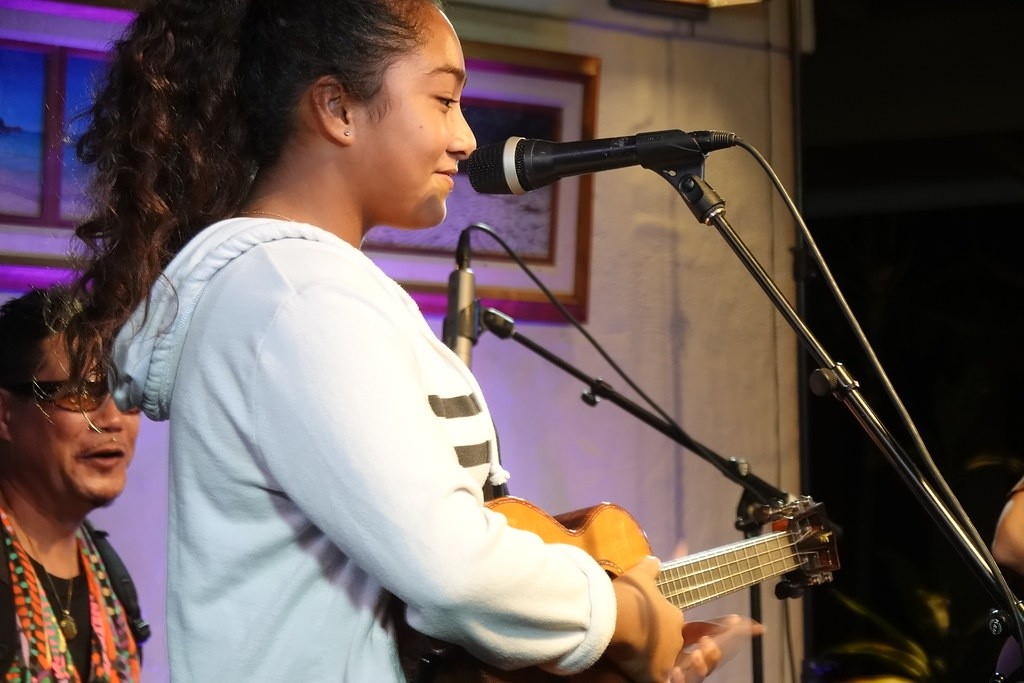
[989,471,1024,682]
[63,3,767,683]
[0,285,150,683]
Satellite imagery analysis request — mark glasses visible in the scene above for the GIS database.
[12,376,111,412]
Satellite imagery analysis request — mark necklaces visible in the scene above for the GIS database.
[7,497,82,642]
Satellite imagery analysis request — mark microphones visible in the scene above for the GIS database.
[445,268,475,372]
[468,129,736,195]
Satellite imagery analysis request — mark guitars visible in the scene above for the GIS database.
[393,484,843,681]
